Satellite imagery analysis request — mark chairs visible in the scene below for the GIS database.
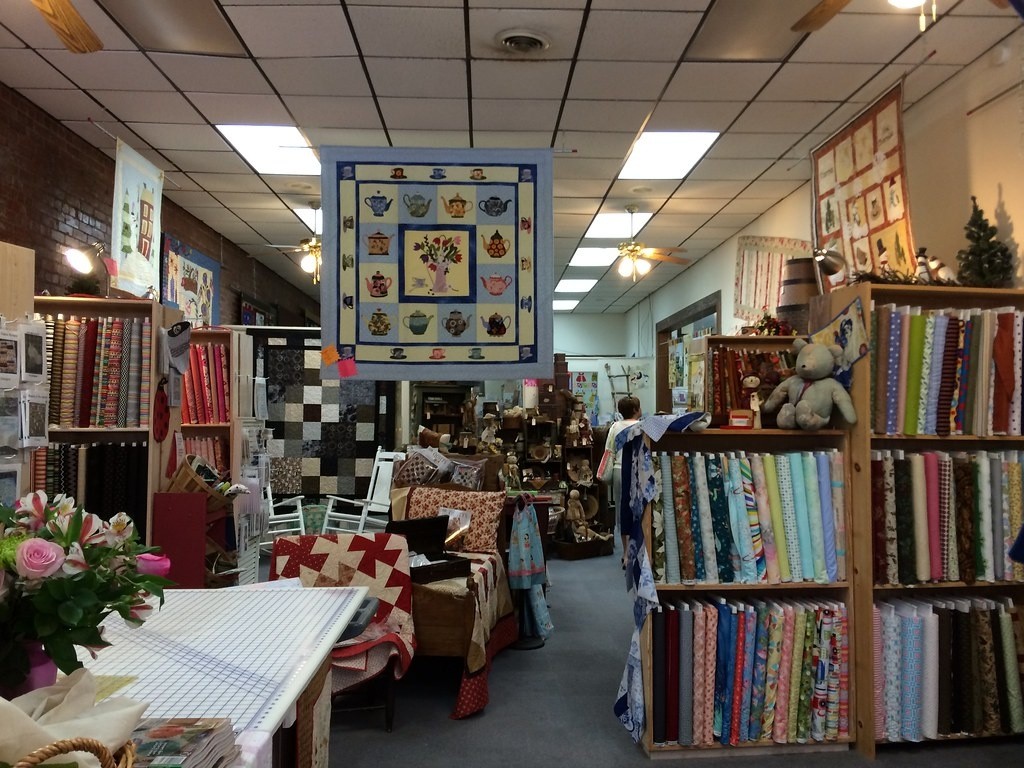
[259,482,306,547]
[268,533,418,733]
[384,484,519,721]
[321,445,406,534]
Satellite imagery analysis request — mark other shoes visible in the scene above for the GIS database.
[621,557,626,570]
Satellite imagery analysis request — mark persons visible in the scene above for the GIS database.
[460,387,594,492]
[566,490,614,541]
[605,396,642,570]
[580,460,593,481]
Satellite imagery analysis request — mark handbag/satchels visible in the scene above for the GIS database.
[596,422,617,481]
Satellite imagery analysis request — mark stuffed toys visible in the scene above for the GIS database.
[764,338,856,430]
[739,370,763,411]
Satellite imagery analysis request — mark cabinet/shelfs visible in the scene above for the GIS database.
[151,492,227,589]
[35,294,186,547]
[412,383,470,438]
[159,329,254,528]
[829,282,1024,762]
[685,334,807,413]
[638,429,858,761]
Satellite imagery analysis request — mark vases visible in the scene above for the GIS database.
[0,641,57,701]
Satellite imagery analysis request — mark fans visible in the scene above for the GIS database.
[615,204,691,264]
[791,0,1010,32]
[265,199,322,254]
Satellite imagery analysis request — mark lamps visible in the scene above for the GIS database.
[811,247,852,285]
[617,256,651,283]
[301,251,322,284]
[65,241,113,299]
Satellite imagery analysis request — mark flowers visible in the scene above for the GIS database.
[0,490,180,690]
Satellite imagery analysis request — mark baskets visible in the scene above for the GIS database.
[13,737,136,768]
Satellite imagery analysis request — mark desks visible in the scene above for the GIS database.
[506,501,561,651]
[42,586,370,768]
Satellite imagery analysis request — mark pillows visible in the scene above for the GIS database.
[416,424,451,454]
[444,453,507,492]
